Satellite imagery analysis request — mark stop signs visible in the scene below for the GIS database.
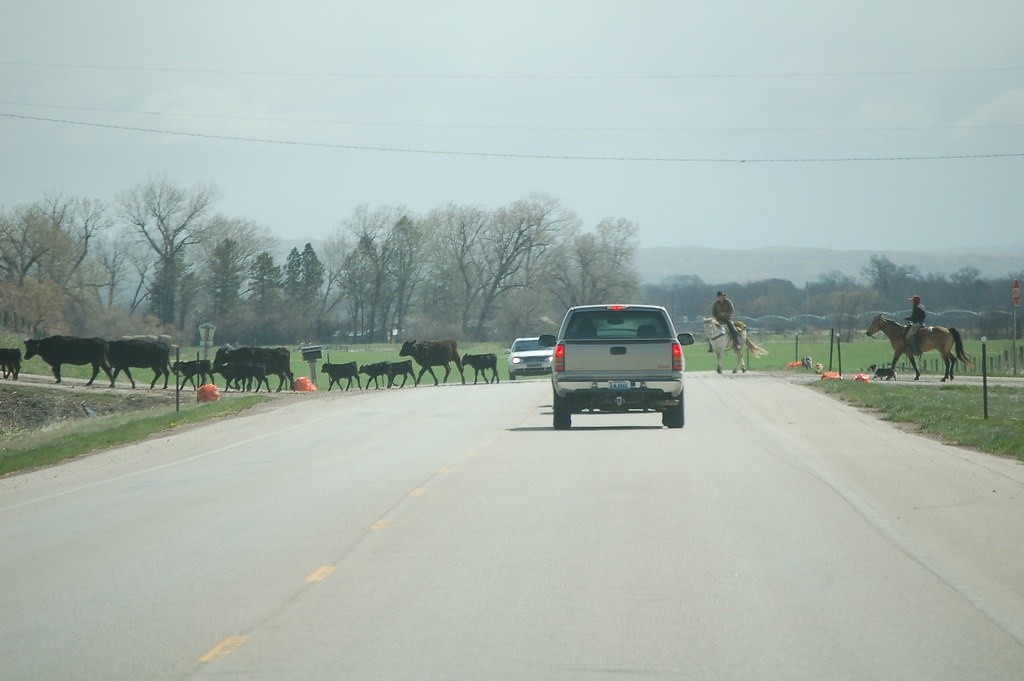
[1013,278,1021,306]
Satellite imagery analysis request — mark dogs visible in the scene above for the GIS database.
[867,365,896,381]
[802,356,823,374]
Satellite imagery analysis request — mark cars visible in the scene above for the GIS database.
[504,337,554,380]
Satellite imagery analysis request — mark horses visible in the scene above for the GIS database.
[702,317,769,374]
[865,313,972,382]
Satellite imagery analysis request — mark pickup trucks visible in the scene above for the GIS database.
[538,303,695,430]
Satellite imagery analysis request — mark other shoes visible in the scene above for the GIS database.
[736,344,741,350]
[708,347,712,352]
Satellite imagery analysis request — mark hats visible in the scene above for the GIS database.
[909,296,921,304]
[717,291,727,297]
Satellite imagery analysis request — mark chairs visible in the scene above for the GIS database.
[577,324,596,336]
[636,325,656,335]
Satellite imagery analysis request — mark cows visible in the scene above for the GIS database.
[0,348,22,381]
[106,339,182,390]
[358,361,417,389]
[24,334,115,388]
[171,359,214,390]
[211,347,295,392]
[461,353,500,383]
[321,361,362,390]
[399,340,465,386]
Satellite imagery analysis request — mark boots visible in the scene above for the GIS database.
[910,341,918,354]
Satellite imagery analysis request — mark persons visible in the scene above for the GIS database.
[708,291,741,352]
[904,295,926,353]
